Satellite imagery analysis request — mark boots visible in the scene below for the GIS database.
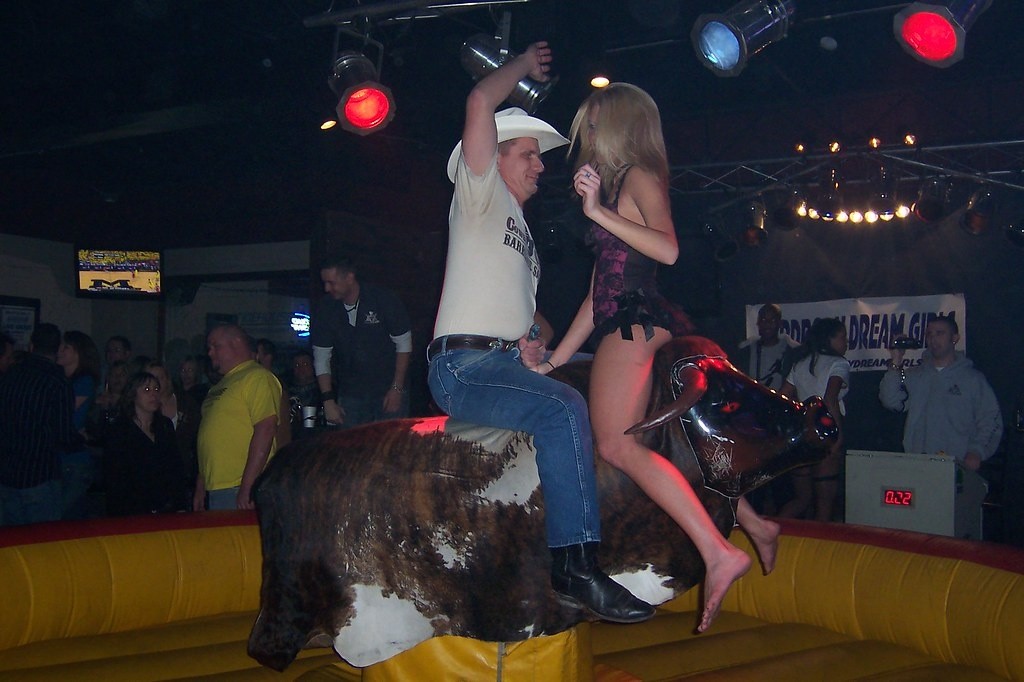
[551,543,657,623]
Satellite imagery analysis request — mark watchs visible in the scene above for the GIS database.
[392,382,406,393]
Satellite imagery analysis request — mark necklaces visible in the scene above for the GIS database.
[345,297,357,311]
[596,161,630,176]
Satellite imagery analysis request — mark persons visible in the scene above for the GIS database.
[529,82,780,634]
[427,39,658,624]
[0,319,327,526]
[772,318,851,521]
[731,303,802,394]
[311,255,413,429]
[878,315,1003,538]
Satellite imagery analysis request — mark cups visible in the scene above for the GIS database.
[302,405,317,428]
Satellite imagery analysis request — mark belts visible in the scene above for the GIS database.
[429,336,515,359]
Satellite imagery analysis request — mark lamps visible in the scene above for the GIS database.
[458,4,560,116]
[893,0,993,69]
[702,127,1024,262]
[326,19,396,136]
[690,0,795,78]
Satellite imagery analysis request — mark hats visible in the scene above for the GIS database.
[447,107,571,182]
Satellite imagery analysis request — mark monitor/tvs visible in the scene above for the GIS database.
[73,244,165,301]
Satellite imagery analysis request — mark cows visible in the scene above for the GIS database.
[244,334,841,673]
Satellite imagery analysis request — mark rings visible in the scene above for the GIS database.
[585,172,591,178]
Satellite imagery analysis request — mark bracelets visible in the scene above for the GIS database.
[546,361,556,370]
[890,362,901,372]
[322,390,336,400]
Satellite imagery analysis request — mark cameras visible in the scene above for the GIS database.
[893,337,922,350]
